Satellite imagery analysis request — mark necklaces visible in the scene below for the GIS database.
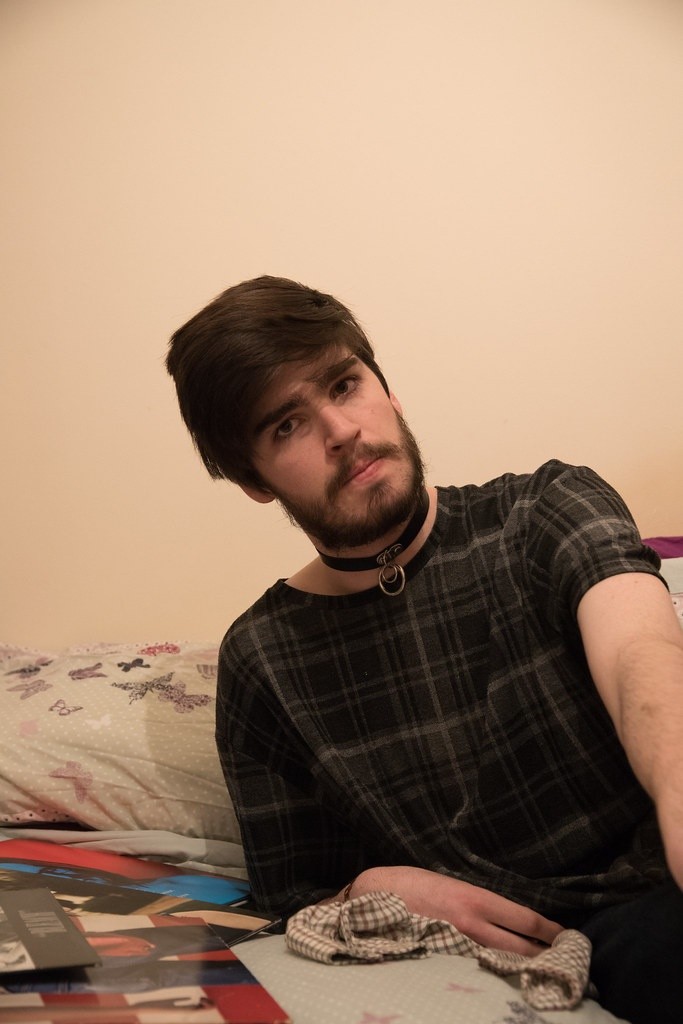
[314,484,431,599]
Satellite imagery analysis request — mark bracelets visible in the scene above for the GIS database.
[344,873,356,901]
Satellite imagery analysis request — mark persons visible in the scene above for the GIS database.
[161,273,683,1024]
